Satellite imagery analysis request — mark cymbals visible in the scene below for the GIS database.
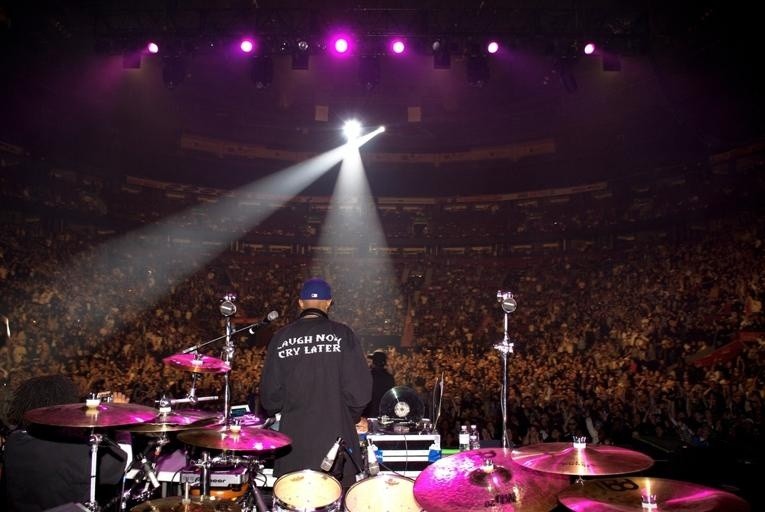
[27,402,162,427]
[558,477,731,512]
[176,421,290,452]
[414,446,571,512]
[144,408,219,427]
[127,495,241,512]
[163,351,232,375]
[512,441,653,477]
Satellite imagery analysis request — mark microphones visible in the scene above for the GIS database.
[501,298,517,313]
[320,437,341,472]
[219,301,237,317]
[248,310,278,335]
[366,445,381,476]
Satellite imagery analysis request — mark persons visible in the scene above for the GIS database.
[259,279,373,494]
[0,374,131,512]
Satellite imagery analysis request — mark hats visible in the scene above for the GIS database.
[367,350,386,361]
[299,278,332,300]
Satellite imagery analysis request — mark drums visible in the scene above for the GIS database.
[343,472,424,511]
[272,468,343,511]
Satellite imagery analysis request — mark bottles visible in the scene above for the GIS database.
[459,425,481,452]
